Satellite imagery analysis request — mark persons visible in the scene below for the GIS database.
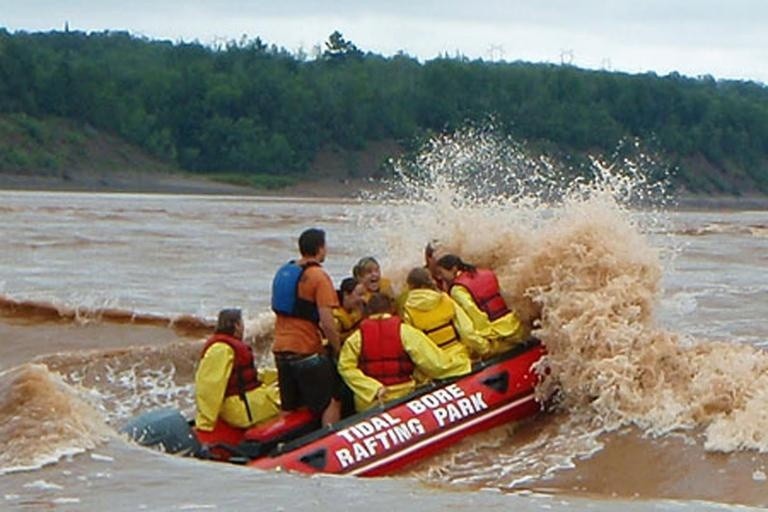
[323,239,527,429]
[194,305,280,434]
[273,228,345,429]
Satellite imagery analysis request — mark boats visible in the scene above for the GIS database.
[117,342,548,478]
